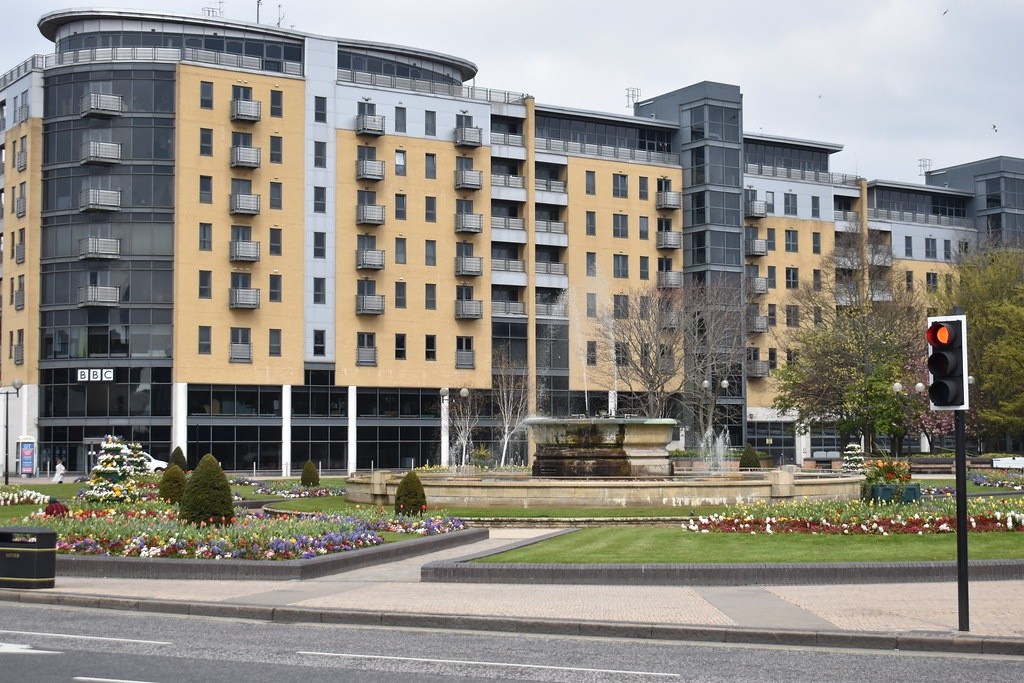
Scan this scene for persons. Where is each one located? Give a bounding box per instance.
[52,460,65,484]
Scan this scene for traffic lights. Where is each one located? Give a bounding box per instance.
[924,315,969,410]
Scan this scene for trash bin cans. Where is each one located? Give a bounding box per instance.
[0,526,57,589]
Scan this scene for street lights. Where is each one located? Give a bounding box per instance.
[0,379,22,485]
[721,378,729,429]
[915,382,926,438]
[460,388,469,417]
[702,379,711,430]
[892,382,902,456]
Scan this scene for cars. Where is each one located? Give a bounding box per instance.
[136,451,170,475]
